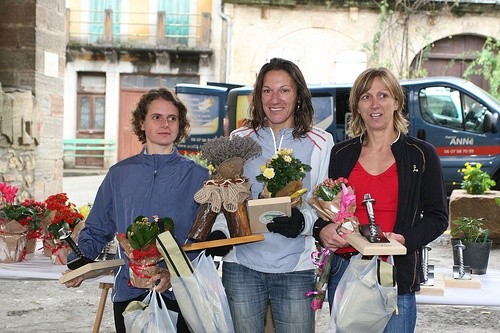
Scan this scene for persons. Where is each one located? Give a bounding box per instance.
[312,66,449,333]
[61,87,213,333]
[206,57,335,333]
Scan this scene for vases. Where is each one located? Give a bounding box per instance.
[0,211,85,264]
[450,189,500,246]
[121,243,160,289]
[279,179,303,208]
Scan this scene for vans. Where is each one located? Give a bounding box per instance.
[174,75,500,198]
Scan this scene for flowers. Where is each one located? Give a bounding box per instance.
[0,183,90,239]
[305,178,361,235]
[453,161,496,194]
[256,148,313,197]
[116,212,176,252]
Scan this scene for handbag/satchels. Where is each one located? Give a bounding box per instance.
[122,283,178,333]
[169,249,235,333]
[330,254,398,333]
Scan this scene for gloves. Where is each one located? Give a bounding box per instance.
[204,230,233,257]
[266,207,306,238]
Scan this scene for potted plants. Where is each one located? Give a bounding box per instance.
[450,217,493,275]
